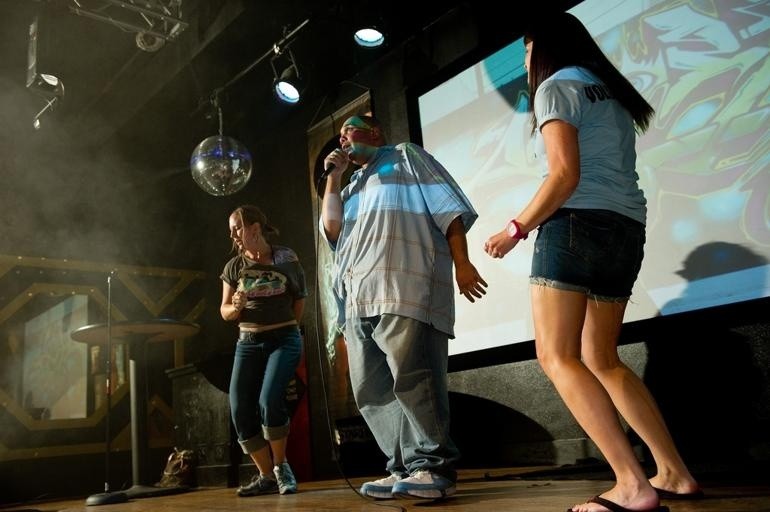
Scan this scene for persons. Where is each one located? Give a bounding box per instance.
[318,113,488,501]
[218,204,308,496]
[483,14,705,512]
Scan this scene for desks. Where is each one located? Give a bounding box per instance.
[69,312,204,500]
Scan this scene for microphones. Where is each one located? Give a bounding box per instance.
[107,268,118,280]
[321,149,346,179]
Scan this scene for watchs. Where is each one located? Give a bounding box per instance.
[505,219,530,240]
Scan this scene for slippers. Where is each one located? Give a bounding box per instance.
[652,484,702,501]
[567,495,669,512]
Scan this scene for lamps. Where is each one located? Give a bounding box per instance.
[269,37,318,105]
[352,0,388,48]
[26,2,73,100]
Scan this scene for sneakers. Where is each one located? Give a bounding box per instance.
[236,476,278,497]
[391,471,456,499]
[360,474,400,498]
[272,463,297,495]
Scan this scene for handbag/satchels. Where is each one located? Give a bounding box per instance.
[160,450,197,489]
